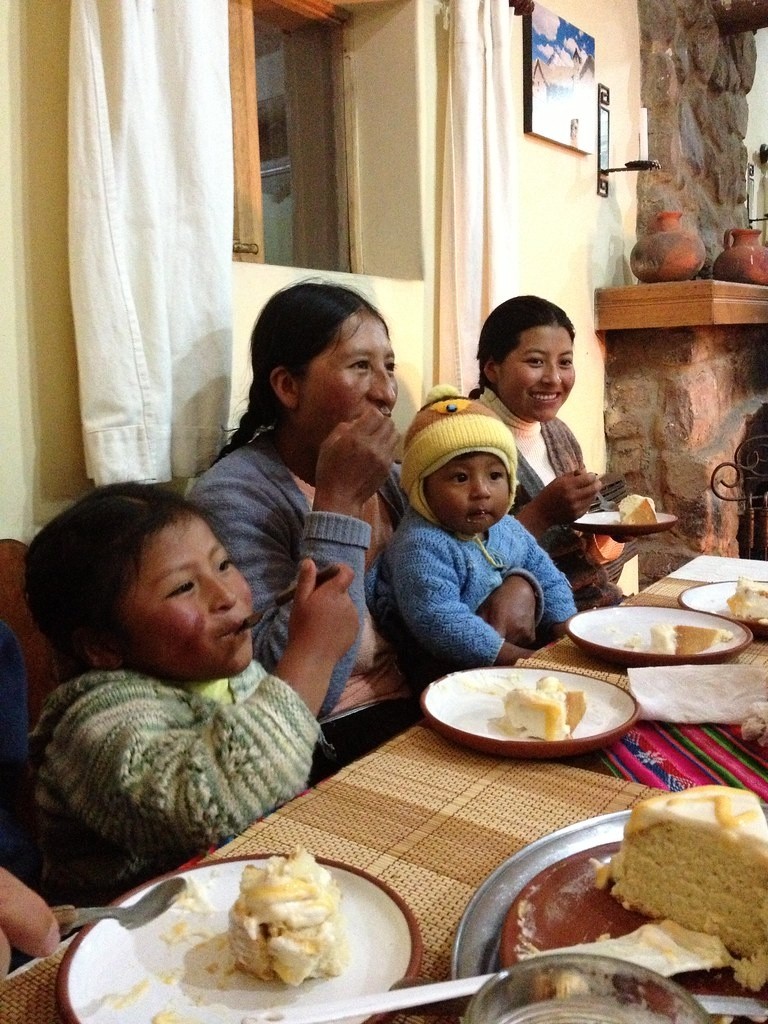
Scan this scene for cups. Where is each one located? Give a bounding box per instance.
[464,952,711,1024]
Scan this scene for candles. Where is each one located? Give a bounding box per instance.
[639,107,649,161]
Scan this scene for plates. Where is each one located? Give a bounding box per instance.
[500,840,768,1024]
[566,604,753,667]
[53,853,422,1024]
[419,665,642,759]
[677,581,768,638]
[571,511,678,536]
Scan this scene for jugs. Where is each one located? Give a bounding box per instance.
[713,228,768,286]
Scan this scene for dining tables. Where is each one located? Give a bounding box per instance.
[0,555,768,1024]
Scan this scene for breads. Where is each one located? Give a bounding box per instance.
[226,845,346,985]
[500,675,587,741]
[595,785,768,992]
[648,623,733,654]
[618,493,658,525]
[726,576,768,620]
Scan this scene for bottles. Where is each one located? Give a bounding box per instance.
[629,212,706,285]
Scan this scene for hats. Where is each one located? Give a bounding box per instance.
[400,383,518,538]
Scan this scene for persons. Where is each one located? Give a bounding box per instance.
[1,619,61,986]
[384,383,579,672]
[181,273,545,790]
[467,295,660,612]
[17,480,358,911]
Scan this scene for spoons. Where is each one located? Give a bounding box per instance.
[573,470,617,512]
[51,877,187,930]
[235,566,340,633]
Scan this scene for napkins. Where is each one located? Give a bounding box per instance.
[627,665,768,724]
